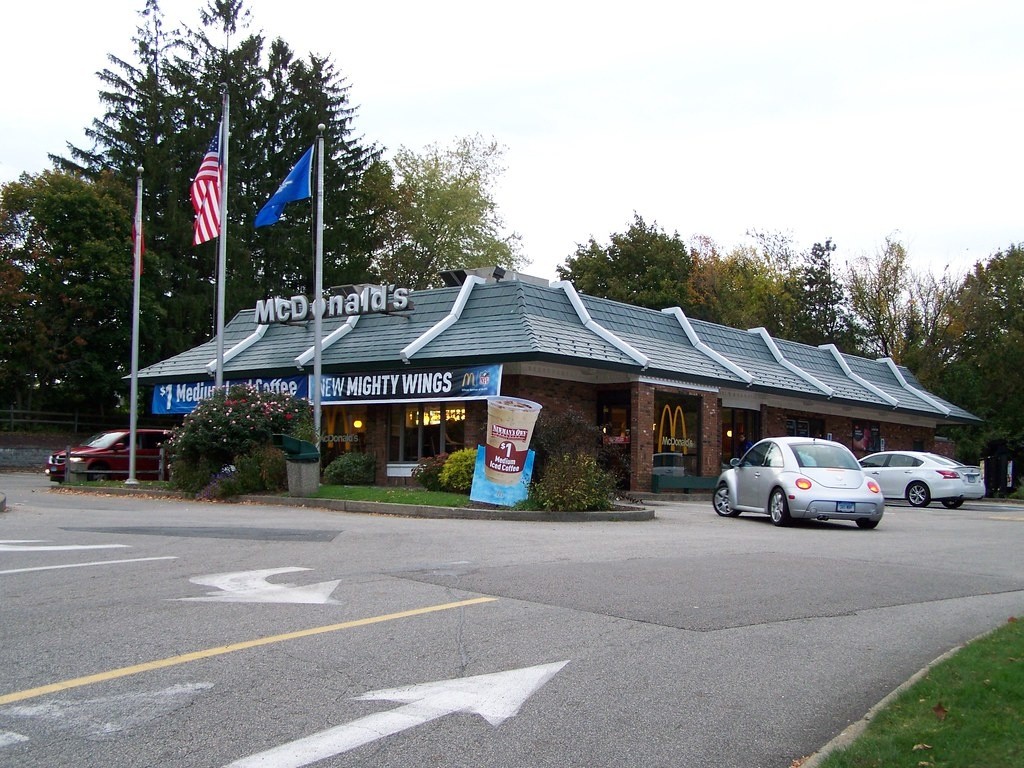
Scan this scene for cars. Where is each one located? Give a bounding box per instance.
[44,428,174,484]
[857,449,987,509]
[710,436,886,529]
[651,452,685,478]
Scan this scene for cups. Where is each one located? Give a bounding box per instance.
[485,397,543,486]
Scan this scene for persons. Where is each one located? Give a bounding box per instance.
[738,433,757,466]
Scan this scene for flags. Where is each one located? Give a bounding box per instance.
[132,196,145,282]
[191,94,226,246]
[254,143,314,228]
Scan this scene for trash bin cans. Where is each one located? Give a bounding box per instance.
[272,434,322,497]
